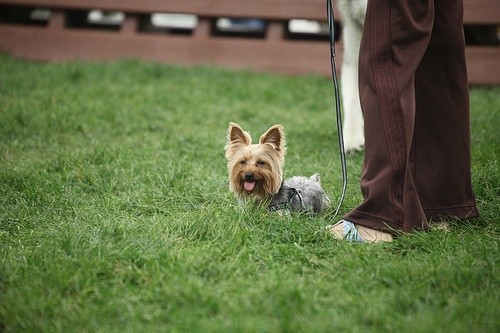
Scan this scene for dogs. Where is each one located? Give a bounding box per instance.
[224,120,331,223]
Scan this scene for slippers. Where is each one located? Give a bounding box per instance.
[325,219,393,243]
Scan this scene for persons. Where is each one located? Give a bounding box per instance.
[324,0,480,245]
